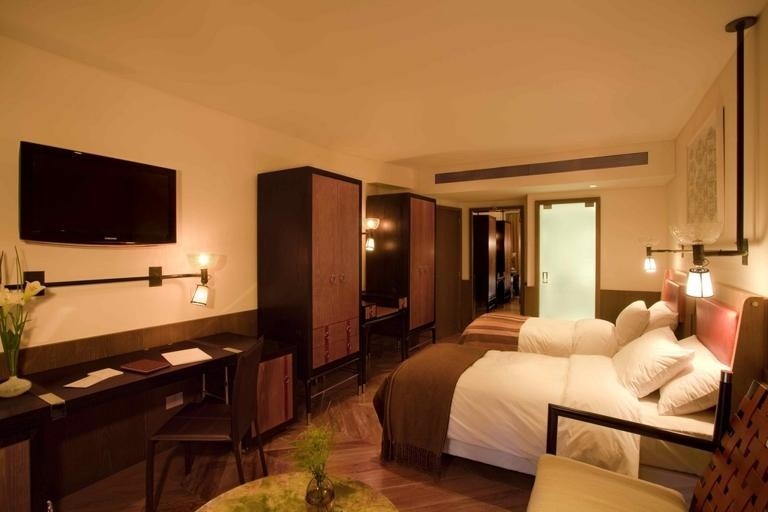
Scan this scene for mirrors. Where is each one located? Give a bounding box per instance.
[468,203,526,317]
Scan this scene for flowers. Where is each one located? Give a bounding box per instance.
[0,245,47,398]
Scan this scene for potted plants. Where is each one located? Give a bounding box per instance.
[295,423,338,512]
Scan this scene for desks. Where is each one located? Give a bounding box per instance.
[0,331,264,512]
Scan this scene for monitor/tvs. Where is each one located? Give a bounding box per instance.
[17,141,176,245]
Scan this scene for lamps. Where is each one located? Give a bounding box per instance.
[641,245,692,273]
[361,216,381,253]
[685,237,749,299]
[187,252,216,308]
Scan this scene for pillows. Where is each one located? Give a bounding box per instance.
[640,300,680,336]
[615,299,651,345]
[612,326,696,400]
[657,334,732,416]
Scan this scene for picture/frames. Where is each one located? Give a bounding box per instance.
[677,103,725,247]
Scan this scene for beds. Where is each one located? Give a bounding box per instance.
[370,280,768,502]
[456,267,695,353]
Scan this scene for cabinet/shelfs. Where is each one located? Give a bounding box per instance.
[255,164,364,426]
[364,191,438,362]
[208,350,295,446]
[473,214,498,313]
[495,220,514,306]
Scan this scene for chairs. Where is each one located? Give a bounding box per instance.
[360,298,409,394]
[524,369,768,512]
[144,335,269,512]
